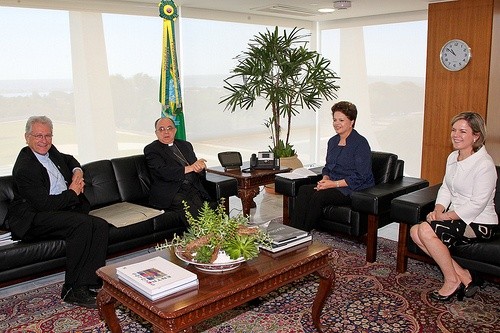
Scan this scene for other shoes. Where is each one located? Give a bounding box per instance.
[61,285,98,308]
[250,296,263,304]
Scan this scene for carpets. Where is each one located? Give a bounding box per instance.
[0,233,500,333]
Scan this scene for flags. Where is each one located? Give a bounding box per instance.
[158,19,186,141]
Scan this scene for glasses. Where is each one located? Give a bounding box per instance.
[27,134,53,140]
[155,127,175,132]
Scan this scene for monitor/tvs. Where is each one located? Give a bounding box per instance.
[218,152,242,166]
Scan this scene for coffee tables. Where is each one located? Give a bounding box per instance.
[95,235,335,333]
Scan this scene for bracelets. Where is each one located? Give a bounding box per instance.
[337,181,339,187]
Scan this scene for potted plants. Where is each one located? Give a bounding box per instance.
[221,25,339,194]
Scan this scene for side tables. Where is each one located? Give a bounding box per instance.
[205,161,293,222]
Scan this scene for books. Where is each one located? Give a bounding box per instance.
[116,256,199,301]
[256,220,312,253]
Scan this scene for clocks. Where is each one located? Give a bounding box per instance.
[440,40,471,71]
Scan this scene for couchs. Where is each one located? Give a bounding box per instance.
[275,149,427,262]
[0,153,237,283]
[391,166,500,280]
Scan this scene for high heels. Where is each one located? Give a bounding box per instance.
[431,282,465,302]
[462,268,484,296]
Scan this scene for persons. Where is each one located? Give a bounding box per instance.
[293,102,374,232]
[143,117,207,235]
[8,116,110,307]
[410,112,500,301]
